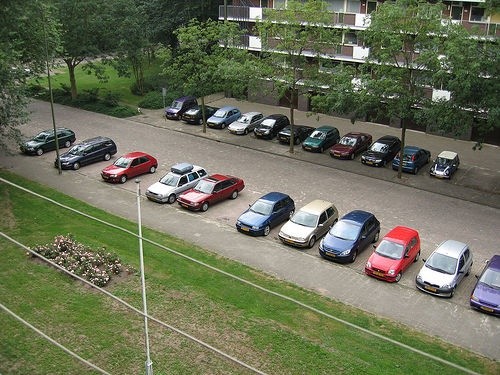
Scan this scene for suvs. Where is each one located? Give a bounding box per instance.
[54,135,118,171]
[165,97,199,120]
[19,127,76,156]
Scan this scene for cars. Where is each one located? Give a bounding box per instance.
[101,152,158,184]
[328,131,373,160]
[302,125,340,154]
[360,135,401,167]
[206,106,241,130]
[278,124,317,146]
[254,113,290,140]
[470,255,500,317]
[144,163,208,204]
[366,225,422,284]
[180,104,220,125]
[278,198,341,250]
[228,112,266,136]
[234,192,296,238]
[175,173,245,213]
[393,145,431,175]
[429,150,460,180]
[415,239,472,300]
[317,210,381,266]
